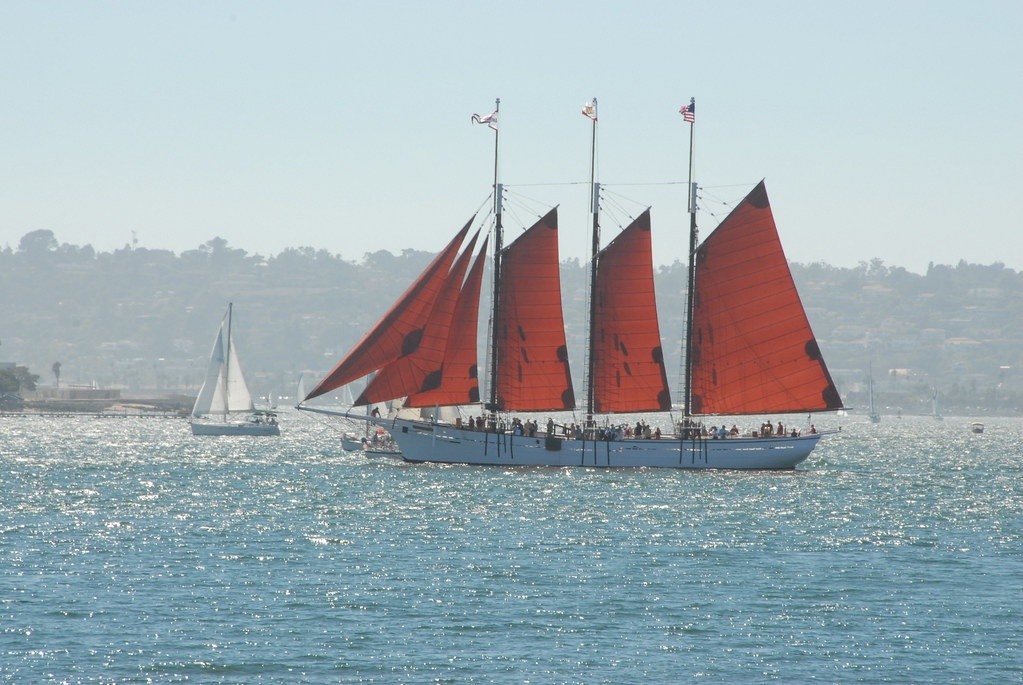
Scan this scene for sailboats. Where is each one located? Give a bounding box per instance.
[295,96,857,472]
[866,361,881,423]
[187,299,288,438]
[338,373,457,463]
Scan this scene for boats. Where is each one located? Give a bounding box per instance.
[970,421,986,434]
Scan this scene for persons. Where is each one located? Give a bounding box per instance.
[634,422,642,438]
[548,418,553,434]
[624,424,634,437]
[512,416,538,436]
[576,425,582,440]
[371,407,380,426]
[373,431,379,441]
[777,421,784,434]
[730,425,738,435]
[641,420,651,438]
[720,425,728,439]
[468,416,474,427]
[791,429,797,437]
[476,416,482,427]
[710,426,718,439]
[811,425,815,434]
[655,428,661,440]
[761,420,773,433]
